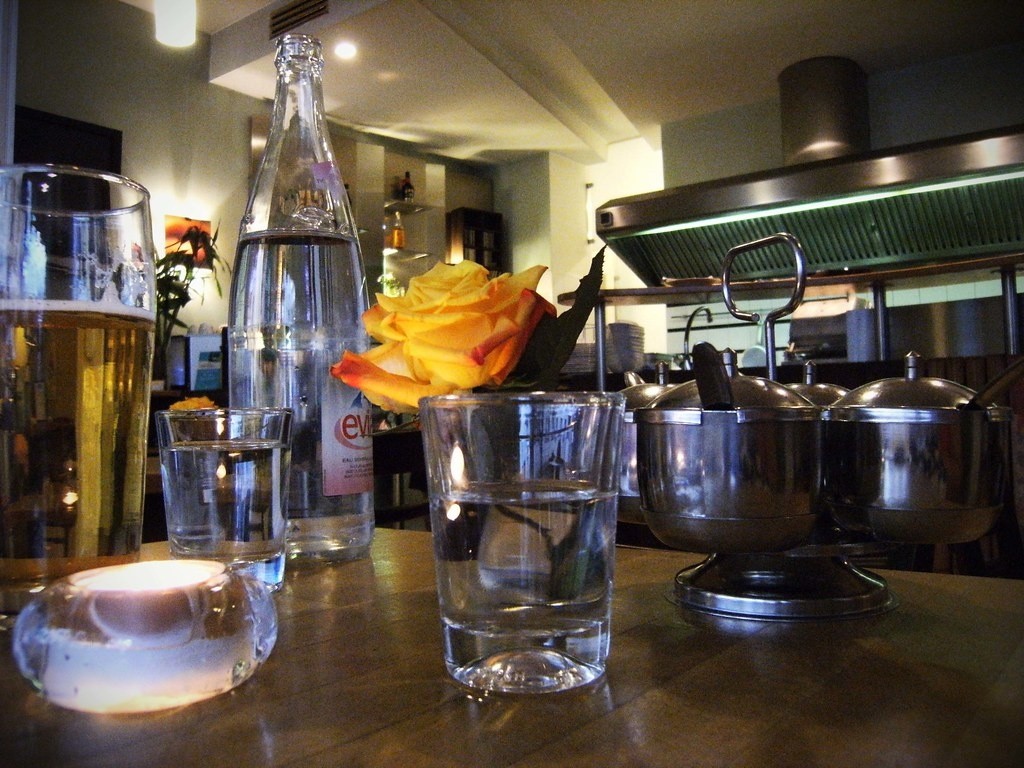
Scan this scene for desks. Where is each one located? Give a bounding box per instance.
[0,524,1024,768]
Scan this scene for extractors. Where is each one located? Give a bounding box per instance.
[595,123,1024,288]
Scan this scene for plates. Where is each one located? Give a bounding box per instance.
[560,342,597,372]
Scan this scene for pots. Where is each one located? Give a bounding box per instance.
[618,361,679,498]
[633,348,821,555]
[822,352,1014,545]
[784,360,850,411]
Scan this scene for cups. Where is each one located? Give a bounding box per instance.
[418,392,627,693]
[161,411,296,592]
[0,163,157,633]
[392,473,411,505]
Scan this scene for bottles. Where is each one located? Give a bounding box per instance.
[402,171,414,203]
[344,184,352,209]
[382,216,393,248]
[228,33,374,561]
[392,211,404,248]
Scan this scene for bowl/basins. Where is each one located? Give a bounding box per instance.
[605,323,644,371]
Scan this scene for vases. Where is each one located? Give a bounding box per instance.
[478,408,612,606]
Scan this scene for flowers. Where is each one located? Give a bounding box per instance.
[169,398,219,413]
[329,245,596,570]
